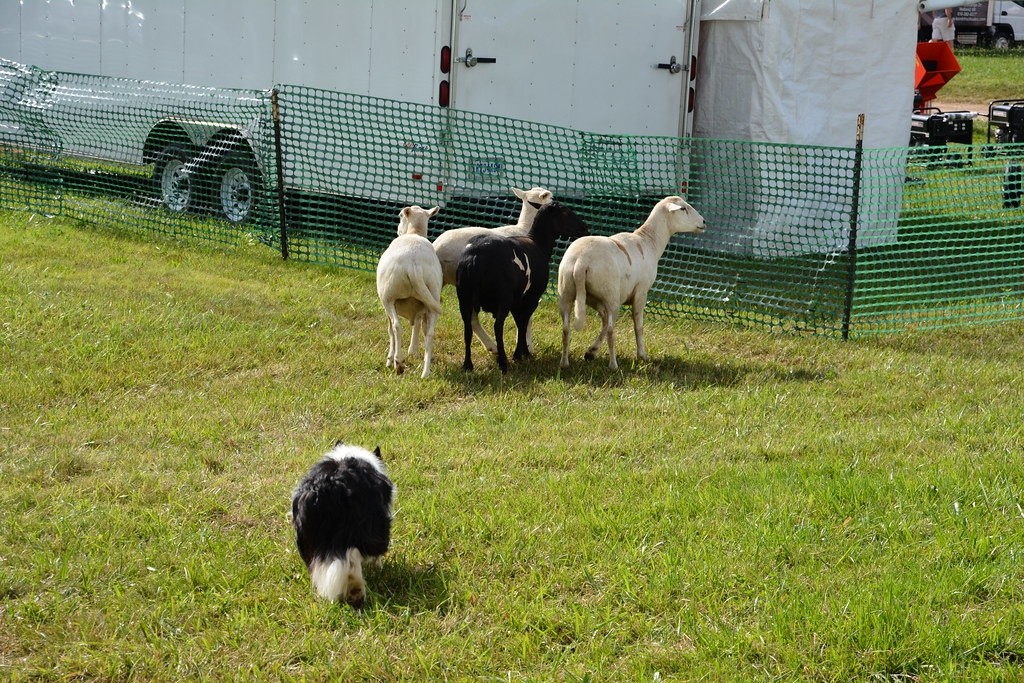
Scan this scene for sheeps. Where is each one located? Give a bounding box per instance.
[557,195,707,384]
[422,185,555,364]
[454,200,590,375]
[376,205,443,380]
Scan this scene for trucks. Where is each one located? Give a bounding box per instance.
[918,0,1024,55]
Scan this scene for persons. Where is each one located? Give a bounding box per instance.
[928,8,956,54]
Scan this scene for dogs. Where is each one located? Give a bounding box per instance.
[292,439,396,608]
